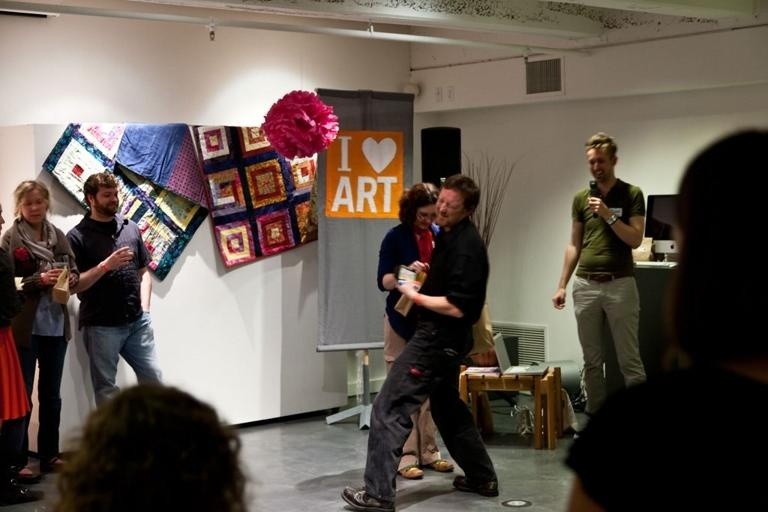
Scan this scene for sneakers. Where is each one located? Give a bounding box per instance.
[342,487,395,512]
[432,458,454,472]
[400,466,424,479]
[453,475,499,497]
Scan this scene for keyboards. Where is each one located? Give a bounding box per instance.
[634,260,676,266]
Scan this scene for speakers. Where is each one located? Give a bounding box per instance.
[420,126,461,180]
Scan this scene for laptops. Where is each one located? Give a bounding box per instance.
[492,333,549,376]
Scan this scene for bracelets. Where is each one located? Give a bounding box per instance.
[413,293,422,304]
[607,214,617,225]
[99,261,109,272]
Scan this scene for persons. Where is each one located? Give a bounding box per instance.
[0,180,80,483]
[552,130,647,415]
[340,175,499,512]
[66,173,162,406]
[52,382,249,512]
[565,123,768,511]
[0,204,44,507]
[377,182,454,479]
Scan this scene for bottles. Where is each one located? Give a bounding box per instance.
[397,279,422,292]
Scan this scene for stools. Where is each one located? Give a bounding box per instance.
[456,365,563,453]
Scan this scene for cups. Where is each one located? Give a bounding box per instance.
[123,249,134,263]
[52,262,66,269]
[417,272,427,283]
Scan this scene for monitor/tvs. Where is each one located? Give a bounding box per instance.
[644,194,681,254]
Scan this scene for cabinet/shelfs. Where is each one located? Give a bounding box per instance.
[605,265,681,396]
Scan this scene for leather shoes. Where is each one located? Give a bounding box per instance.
[1,468,43,506]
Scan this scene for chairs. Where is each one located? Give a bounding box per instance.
[489,333,589,434]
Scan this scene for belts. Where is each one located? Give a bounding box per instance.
[579,272,625,283]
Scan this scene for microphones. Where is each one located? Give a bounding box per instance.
[589,181,599,219]
